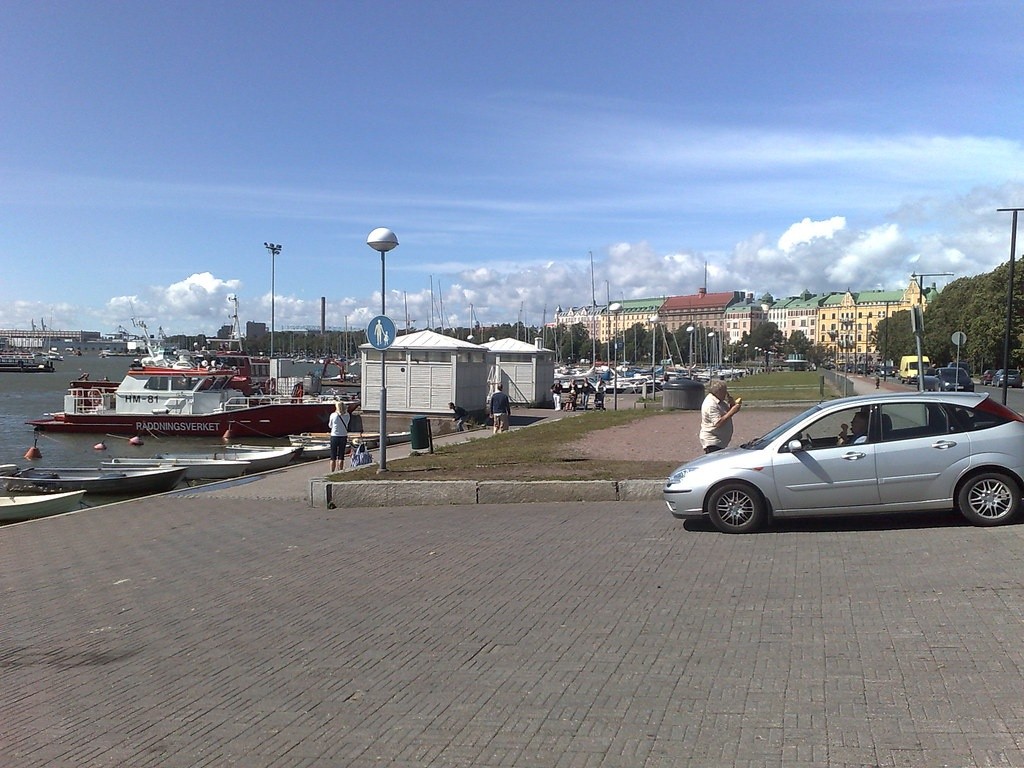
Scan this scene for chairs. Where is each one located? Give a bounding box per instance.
[882,413,893,439]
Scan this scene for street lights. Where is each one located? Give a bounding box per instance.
[708,332,715,390]
[650,316,660,400]
[755,346,759,362]
[996,208,1024,409]
[361,227,401,484]
[609,303,624,410]
[743,343,748,373]
[686,325,695,382]
[263,240,282,358]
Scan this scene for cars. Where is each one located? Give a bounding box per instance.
[979,370,999,386]
[992,369,1023,388]
[823,355,974,393]
[662,390,1024,534]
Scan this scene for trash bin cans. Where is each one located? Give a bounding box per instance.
[409,414,430,449]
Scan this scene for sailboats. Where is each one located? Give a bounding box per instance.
[514,250,751,394]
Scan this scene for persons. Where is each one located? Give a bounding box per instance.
[927,363,936,391]
[596,378,606,408]
[581,378,597,411]
[663,373,669,382]
[875,373,880,389]
[490,384,511,434]
[699,380,741,455]
[329,402,350,472]
[1017,365,1022,375]
[569,378,580,409]
[449,403,471,431]
[348,439,361,466]
[551,380,563,411]
[807,412,868,448]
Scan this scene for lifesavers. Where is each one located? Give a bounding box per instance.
[88,389,102,403]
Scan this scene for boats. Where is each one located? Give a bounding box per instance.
[100,457,252,479]
[286,430,413,450]
[159,447,303,473]
[0,347,65,372]
[0,489,89,523]
[24,300,362,439]
[321,372,362,388]
[0,463,189,495]
[98,351,362,366]
[225,443,352,460]
